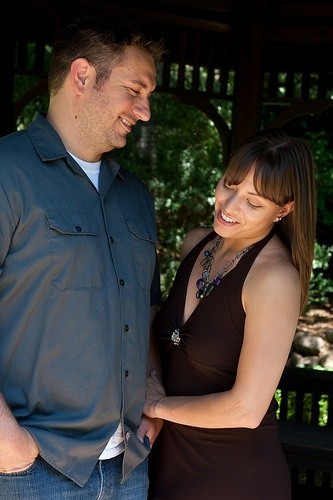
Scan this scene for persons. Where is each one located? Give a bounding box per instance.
[0,9,167,500]
[144,127,316,500]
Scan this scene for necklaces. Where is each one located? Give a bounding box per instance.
[195,234,258,300]
